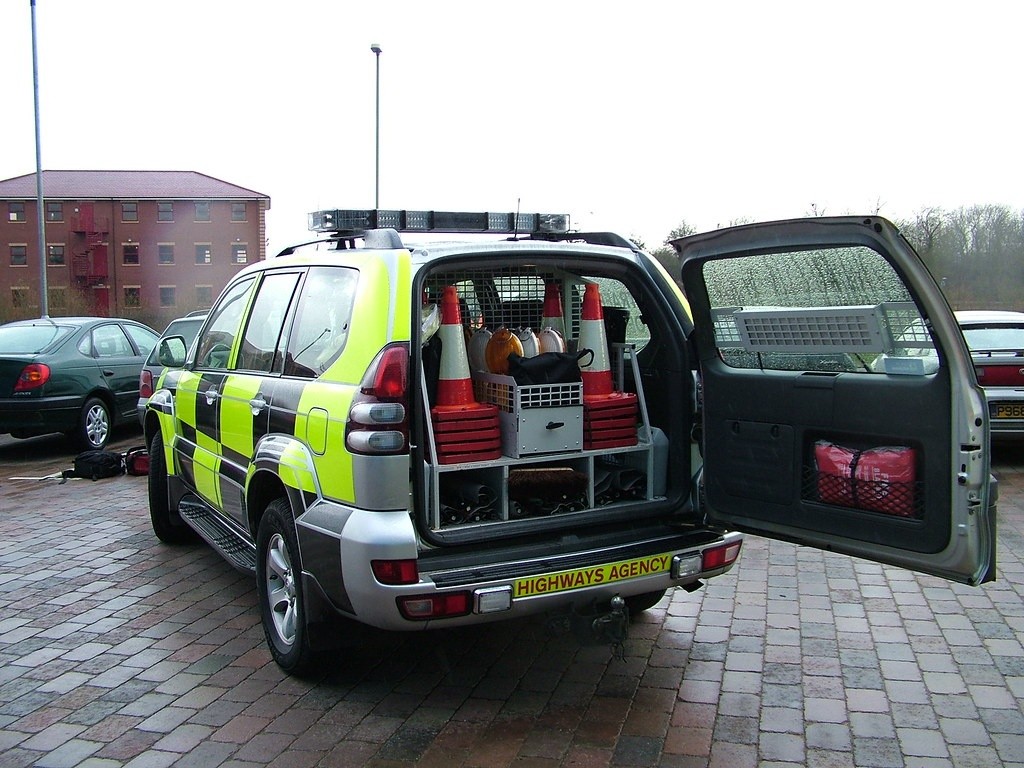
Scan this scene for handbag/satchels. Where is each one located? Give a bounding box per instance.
[125,446,150,476]
[506,348,594,409]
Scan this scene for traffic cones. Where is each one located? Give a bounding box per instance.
[430,283,640,466]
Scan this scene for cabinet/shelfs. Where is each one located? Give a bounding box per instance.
[421,341,655,534]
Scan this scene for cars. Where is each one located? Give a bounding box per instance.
[0,317,162,452]
[849,310,1024,460]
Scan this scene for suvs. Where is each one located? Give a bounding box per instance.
[144,210,997,677]
[136,309,211,426]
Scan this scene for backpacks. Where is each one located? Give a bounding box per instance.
[58,450,126,484]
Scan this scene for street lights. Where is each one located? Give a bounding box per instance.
[371,43,382,211]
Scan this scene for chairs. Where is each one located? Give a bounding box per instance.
[95,336,117,358]
